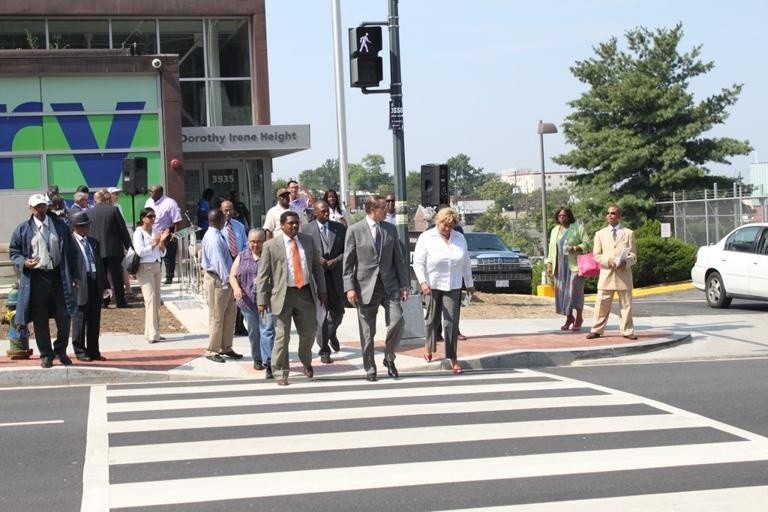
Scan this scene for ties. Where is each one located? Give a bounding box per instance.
[375,223,381,257]
[290,239,304,290]
[82,238,97,280]
[322,225,328,272]
[227,222,238,257]
[40,223,49,266]
[612,228,617,242]
[221,235,226,242]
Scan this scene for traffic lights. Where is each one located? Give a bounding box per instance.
[345,22,392,90]
[347,26,386,88]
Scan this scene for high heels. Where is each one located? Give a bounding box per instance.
[573,319,583,331]
[424,342,432,362]
[450,361,462,373]
[561,317,575,331]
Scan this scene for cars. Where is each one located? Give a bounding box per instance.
[463,232,531,292]
[690,223,768,309]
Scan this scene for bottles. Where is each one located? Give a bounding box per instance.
[563,240,569,255]
[463,292,471,304]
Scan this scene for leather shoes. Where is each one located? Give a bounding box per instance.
[367,373,376,381]
[330,336,339,351]
[277,379,288,385]
[164,278,172,284]
[624,334,637,340]
[90,356,105,361]
[587,333,600,339]
[220,351,243,359]
[265,367,274,379]
[56,352,72,365]
[383,358,398,378]
[304,365,313,378]
[457,334,467,340]
[321,352,330,363]
[76,353,92,362]
[254,360,262,370]
[206,355,225,363]
[40,356,53,368]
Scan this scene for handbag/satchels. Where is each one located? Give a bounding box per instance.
[420,290,435,326]
[121,229,144,275]
[198,206,212,219]
[577,242,600,277]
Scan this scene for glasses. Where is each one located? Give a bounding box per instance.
[386,200,395,203]
[146,215,156,218]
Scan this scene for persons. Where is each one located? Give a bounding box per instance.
[413,206,473,374]
[300,202,349,363]
[384,193,395,222]
[134,208,170,344]
[220,199,250,336]
[266,187,308,233]
[343,195,409,382]
[324,188,345,222]
[547,205,590,332]
[87,189,134,309]
[107,186,124,215]
[50,195,69,217]
[9,195,81,368]
[202,209,243,365]
[196,188,216,240]
[101,189,133,299]
[232,191,254,228]
[70,212,114,363]
[427,203,468,342]
[255,212,318,386]
[228,227,276,378]
[586,205,637,341]
[44,185,59,203]
[77,186,94,211]
[287,181,317,211]
[71,192,90,210]
[145,185,182,284]
[264,228,275,238]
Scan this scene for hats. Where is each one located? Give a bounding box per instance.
[107,187,122,193]
[277,188,291,197]
[29,194,48,207]
[71,212,92,226]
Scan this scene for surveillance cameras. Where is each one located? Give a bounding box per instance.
[152,58,161,69]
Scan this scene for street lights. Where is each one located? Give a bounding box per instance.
[537,114,558,259]
[539,120,560,256]
